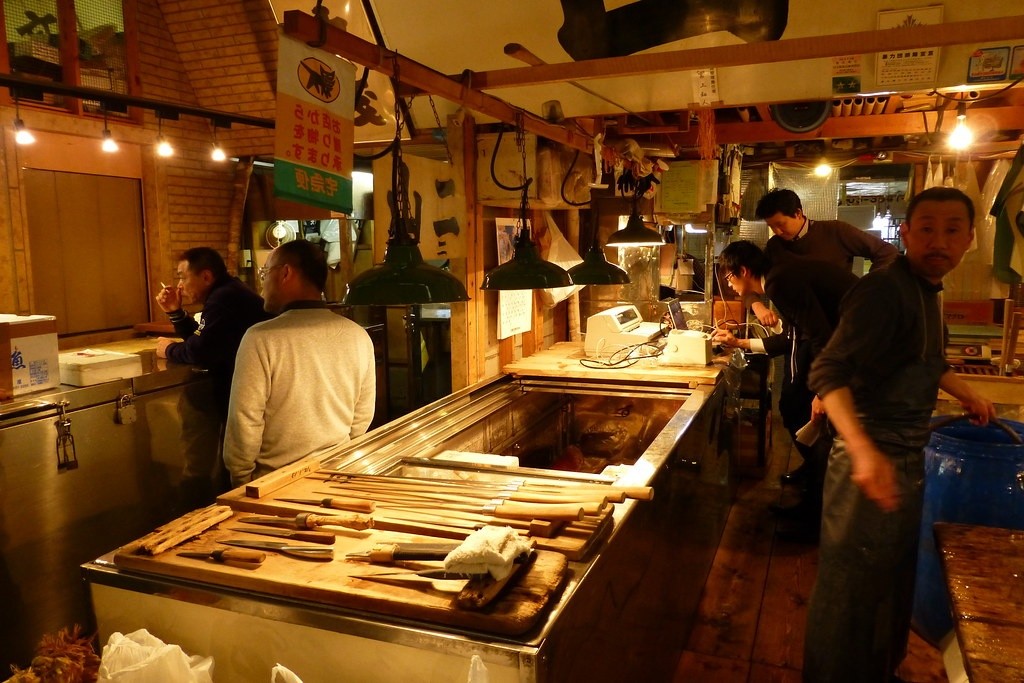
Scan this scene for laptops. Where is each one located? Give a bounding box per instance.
[668,297,726,355]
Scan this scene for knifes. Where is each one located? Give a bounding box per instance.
[228,528,335,544]
[237,513,374,530]
[347,565,484,581]
[217,541,334,559]
[176,548,266,563]
[274,499,376,513]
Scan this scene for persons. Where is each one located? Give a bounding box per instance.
[222,240,376,488]
[802,187,998,683]
[710,240,791,357]
[156,247,279,421]
[743,188,899,486]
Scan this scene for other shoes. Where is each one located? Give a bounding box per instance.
[780,472,804,486]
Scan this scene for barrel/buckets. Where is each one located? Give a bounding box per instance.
[910,414,1024,649]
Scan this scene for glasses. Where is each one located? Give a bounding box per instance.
[723,265,740,281]
[257,263,284,281]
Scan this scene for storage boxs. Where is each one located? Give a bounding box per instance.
[0,313,60,400]
[59,349,143,387]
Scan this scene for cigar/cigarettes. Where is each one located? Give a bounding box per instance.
[160,281,170,294]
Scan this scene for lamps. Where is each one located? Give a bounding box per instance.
[479,120,574,290]
[605,179,666,246]
[568,155,630,285]
[343,67,472,304]
